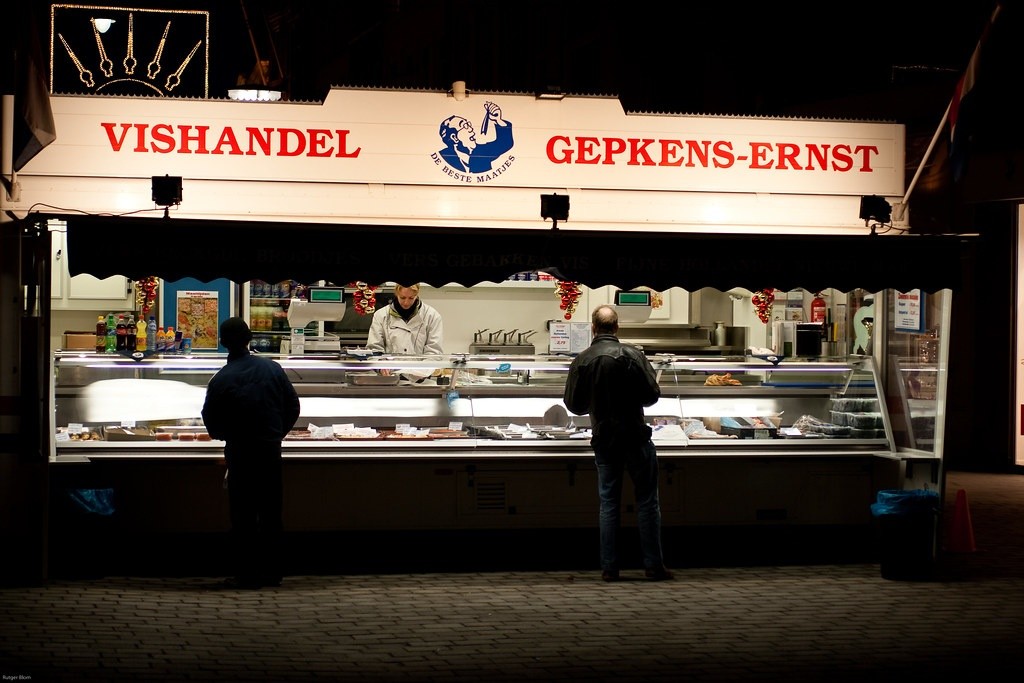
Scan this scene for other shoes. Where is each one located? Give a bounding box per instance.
[222,575,283,590]
[601,567,621,582]
[644,565,673,582]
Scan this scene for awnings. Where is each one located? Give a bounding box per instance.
[20,211,980,299]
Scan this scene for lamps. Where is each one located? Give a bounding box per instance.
[539,194,570,230]
[152,174,184,222]
[860,194,892,236]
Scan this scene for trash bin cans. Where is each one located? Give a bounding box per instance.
[54,487,115,571]
[877,489,939,581]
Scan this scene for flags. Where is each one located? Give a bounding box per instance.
[947,18,989,145]
[11,0,56,173]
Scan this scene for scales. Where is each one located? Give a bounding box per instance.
[280,287,346,354]
[603,291,652,323]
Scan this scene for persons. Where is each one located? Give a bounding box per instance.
[564,305,675,582]
[202,317,300,591]
[367,283,446,382]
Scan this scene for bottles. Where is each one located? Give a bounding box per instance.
[95,314,175,354]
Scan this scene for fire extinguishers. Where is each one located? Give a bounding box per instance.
[810,289,829,323]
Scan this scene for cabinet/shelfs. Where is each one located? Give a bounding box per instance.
[46,217,136,311]
